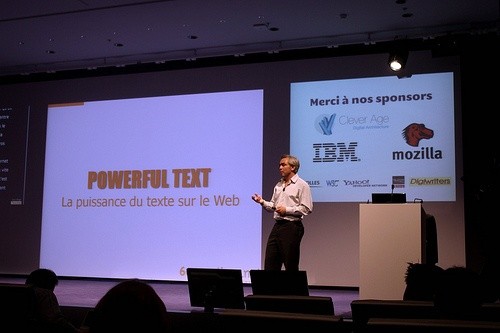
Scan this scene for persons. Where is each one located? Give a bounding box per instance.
[251,155,313,268]
[24,269,79,333]
[403,263,445,301]
[433,267,483,321]
[89,280,167,333]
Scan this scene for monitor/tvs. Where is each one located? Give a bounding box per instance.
[372,192,406,204]
[187,267,245,314]
[250,269,310,297]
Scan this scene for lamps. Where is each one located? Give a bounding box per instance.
[387,44,409,71]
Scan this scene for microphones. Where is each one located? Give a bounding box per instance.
[390,185,395,203]
